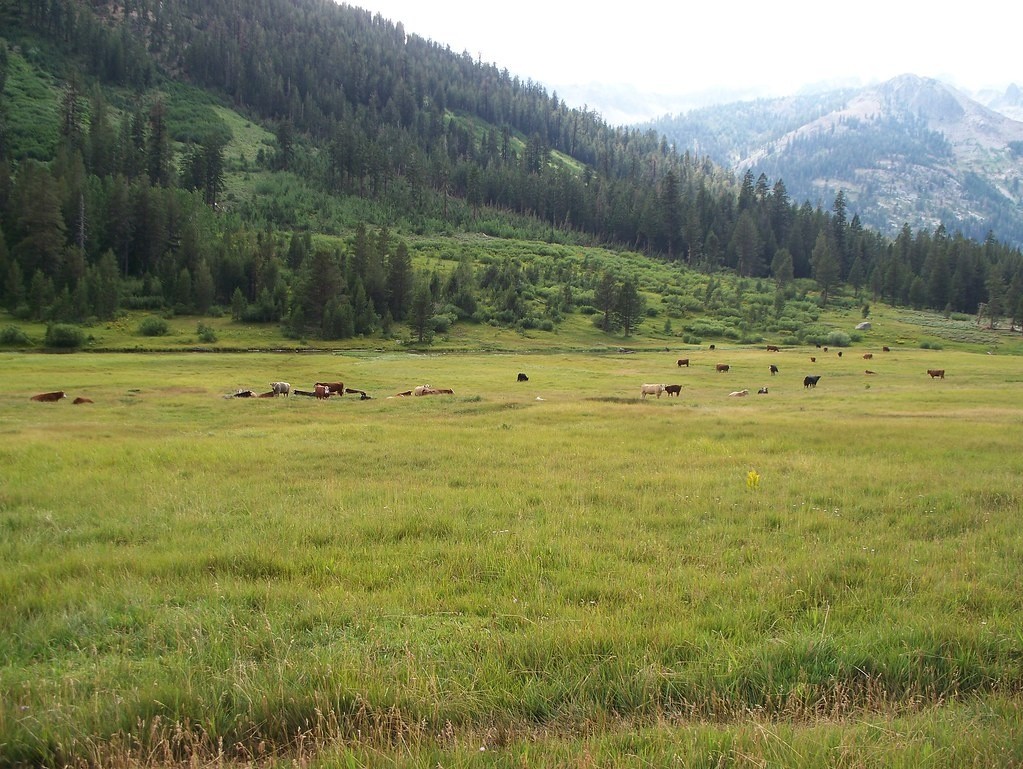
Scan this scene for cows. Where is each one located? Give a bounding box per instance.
[865,370,874,374]
[517,373,528,382]
[676,359,689,367]
[224,388,276,399]
[928,370,944,379]
[768,365,778,376]
[863,346,890,360]
[717,363,732,373]
[71,398,93,404]
[709,345,715,350]
[665,385,682,397]
[297,380,371,400]
[728,387,768,397]
[30,390,66,402]
[767,345,779,352]
[804,376,821,388]
[811,343,842,362]
[641,383,666,399]
[270,382,290,397]
[387,384,454,400]
